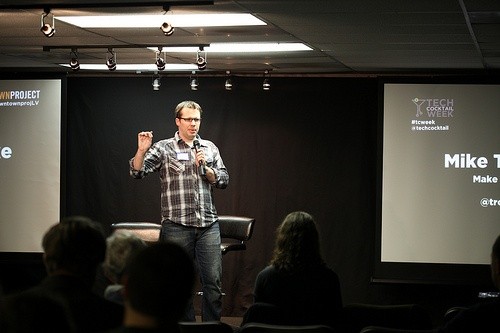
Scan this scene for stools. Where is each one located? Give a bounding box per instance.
[197,216,254,295]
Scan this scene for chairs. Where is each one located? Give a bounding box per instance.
[176,302,469,333]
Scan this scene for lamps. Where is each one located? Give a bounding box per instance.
[69,48,80,73]
[40,8,56,38]
[105,48,117,71]
[263,70,272,91]
[224,71,233,91]
[189,71,200,91]
[196,46,208,71]
[151,72,161,91]
[154,46,167,71]
[161,6,175,36]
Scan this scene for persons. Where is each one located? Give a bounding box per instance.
[129,101,228,322]
[15,217,196,333]
[254,212,346,333]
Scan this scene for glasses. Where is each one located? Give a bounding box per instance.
[178,116,201,123]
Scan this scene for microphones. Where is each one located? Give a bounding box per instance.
[193,140,206,178]
